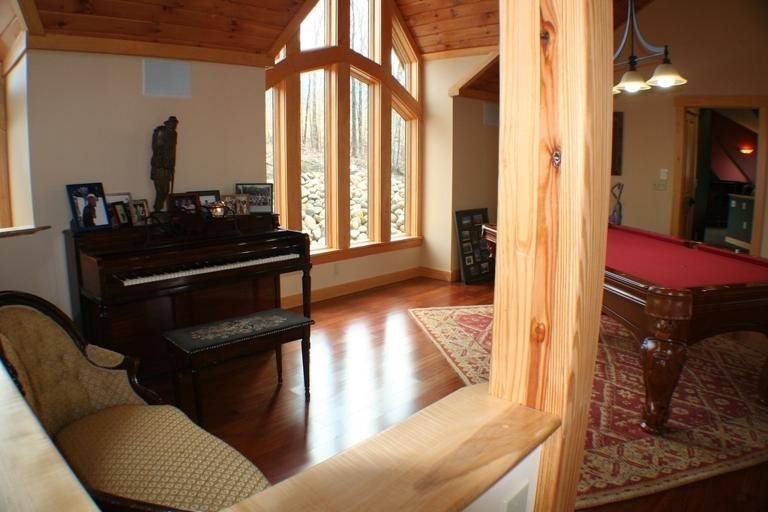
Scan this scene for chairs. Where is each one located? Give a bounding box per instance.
[0,290,275,511]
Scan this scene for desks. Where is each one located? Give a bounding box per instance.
[480,222,768,435]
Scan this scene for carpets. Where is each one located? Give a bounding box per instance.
[406,303,767,509]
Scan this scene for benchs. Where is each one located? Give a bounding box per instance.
[161,307,316,427]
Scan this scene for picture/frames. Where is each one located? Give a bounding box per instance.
[65,182,274,233]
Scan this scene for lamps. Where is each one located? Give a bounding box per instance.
[612,1,689,95]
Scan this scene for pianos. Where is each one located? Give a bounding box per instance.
[63,212,312,348]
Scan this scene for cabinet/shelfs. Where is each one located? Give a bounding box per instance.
[725,192,755,253]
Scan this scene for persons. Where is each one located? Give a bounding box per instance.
[80,194,99,227]
[184,198,196,213]
[174,199,181,212]
[149,116,180,211]
[224,198,248,215]
[139,206,145,220]
[243,191,270,206]
[201,199,211,209]
[179,199,187,212]
